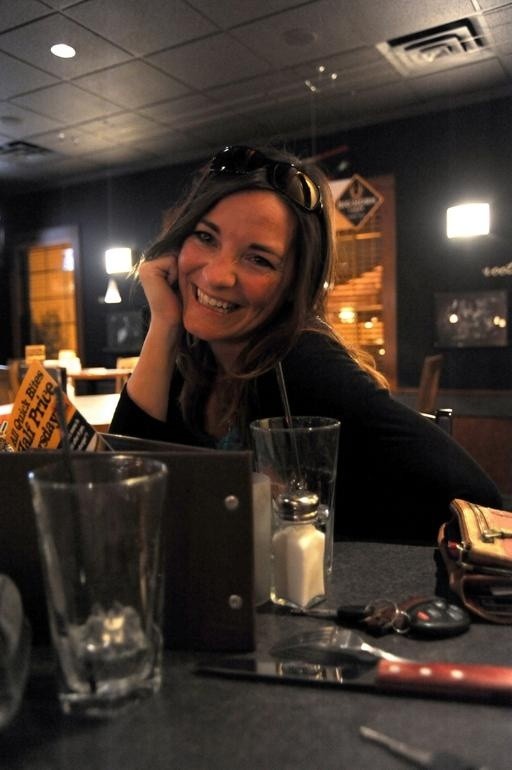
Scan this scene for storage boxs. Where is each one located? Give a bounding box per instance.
[0,432,258,654]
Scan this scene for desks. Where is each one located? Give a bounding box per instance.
[1,391,122,434]
[66,368,133,397]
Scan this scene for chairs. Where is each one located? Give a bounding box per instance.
[116,356,139,395]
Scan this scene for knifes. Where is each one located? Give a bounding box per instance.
[194,657,510,703]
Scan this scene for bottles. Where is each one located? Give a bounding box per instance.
[272,489,327,610]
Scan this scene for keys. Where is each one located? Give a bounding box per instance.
[290,599,469,639]
[359,723,480,769]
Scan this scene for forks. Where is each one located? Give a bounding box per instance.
[271,625,407,663]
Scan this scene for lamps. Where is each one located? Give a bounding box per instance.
[104,162,122,304]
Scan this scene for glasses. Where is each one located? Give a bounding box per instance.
[206,143,322,211]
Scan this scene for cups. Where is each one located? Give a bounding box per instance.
[27,457,169,717]
[250,418,337,608]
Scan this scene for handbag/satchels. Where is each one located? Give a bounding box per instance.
[433,496,512,625]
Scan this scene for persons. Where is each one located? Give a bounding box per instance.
[109,145,504,547]
